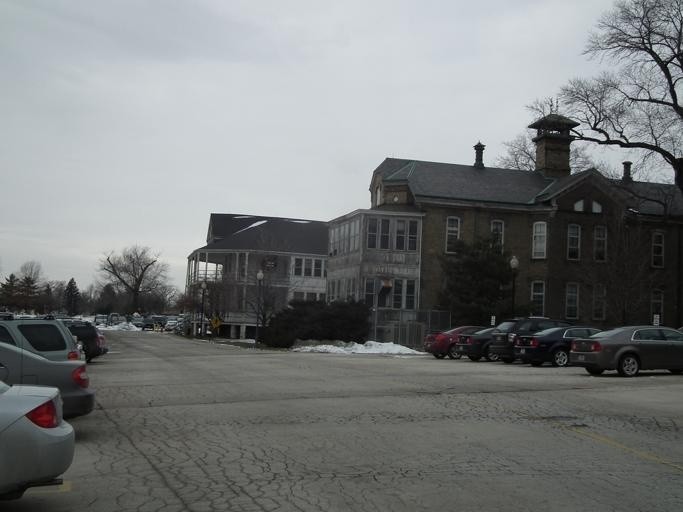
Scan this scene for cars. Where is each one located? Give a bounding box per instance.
[0,316,86,363]
[0,341,96,420]
[61,317,109,364]
[424,316,683,376]
[0,381,78,500]
